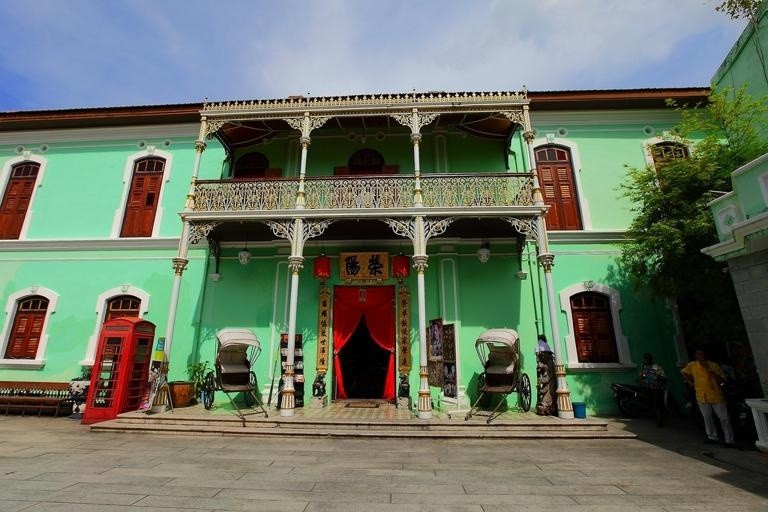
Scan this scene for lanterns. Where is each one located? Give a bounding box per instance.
[391,250,410,284]
[314,249,331,286]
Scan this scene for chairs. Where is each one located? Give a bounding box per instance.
[484,350,515,386]
[219,350,251,385]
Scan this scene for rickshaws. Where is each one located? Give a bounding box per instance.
[461,328,532,423]
[201,327,269,423]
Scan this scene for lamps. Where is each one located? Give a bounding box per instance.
[476,222,491,264]
[238,222,251,265]
[313,233,332,280]
[391,235,410,279]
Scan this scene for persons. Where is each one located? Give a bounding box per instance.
[680,348,737,447]
[430,323,443,355]
[635,354,670,428]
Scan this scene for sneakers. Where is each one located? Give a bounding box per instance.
[724,442,737,449]
[703,439,720,445]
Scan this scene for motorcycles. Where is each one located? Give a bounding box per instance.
[611,382,679,421]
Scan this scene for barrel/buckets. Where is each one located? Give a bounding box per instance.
[572,401,588,419]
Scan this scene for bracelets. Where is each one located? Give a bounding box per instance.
[707,369,711,373]
